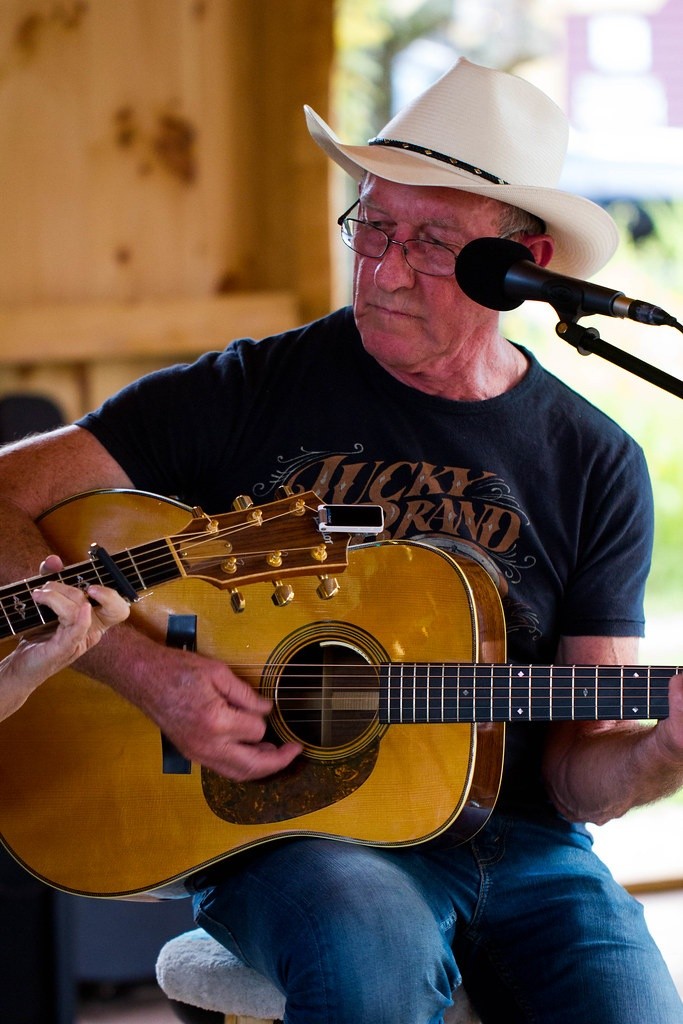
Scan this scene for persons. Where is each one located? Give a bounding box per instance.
[0,60,683,1024]
[0,554,133,727]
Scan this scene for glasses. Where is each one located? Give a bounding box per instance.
[337,199,529,277]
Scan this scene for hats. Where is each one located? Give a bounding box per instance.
[304,56,619,281]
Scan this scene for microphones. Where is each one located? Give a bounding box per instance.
[456,236,670,325]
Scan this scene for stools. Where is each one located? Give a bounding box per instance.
[155,929,288,1024]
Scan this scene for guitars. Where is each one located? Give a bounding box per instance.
[0,482,352,646]
[0,487,683,905]
